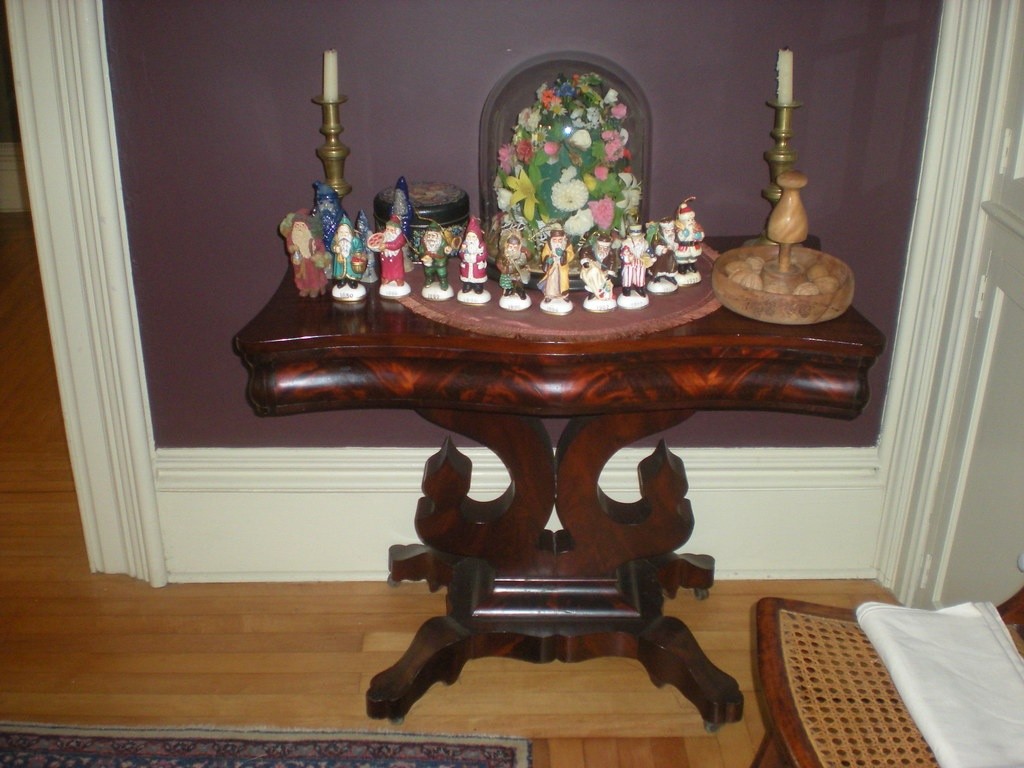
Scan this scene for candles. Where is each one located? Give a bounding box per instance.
[777,46,794,105]
[322,46,339,103]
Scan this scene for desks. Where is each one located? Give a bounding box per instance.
[232,233,887,733]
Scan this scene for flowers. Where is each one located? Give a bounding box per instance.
[494,73,642,251]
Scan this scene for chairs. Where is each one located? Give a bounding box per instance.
[749,582,1024,768]
